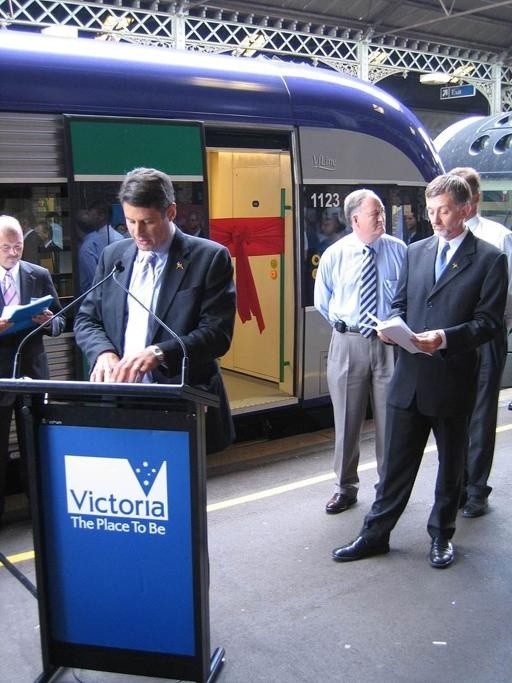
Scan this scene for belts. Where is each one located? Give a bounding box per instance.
[334,322,360,333]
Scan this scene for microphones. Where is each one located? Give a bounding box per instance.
[112,266,190,385]
[13,259,122,379]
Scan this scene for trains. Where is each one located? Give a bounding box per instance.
[2,25,512,468]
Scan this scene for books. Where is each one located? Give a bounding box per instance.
[0,295,53,336]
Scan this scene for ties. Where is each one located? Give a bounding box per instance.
[359,246,378,341]
[123,254,156,384]
[3,271,19,305]
[435,242,451,284]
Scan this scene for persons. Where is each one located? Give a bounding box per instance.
[317,216,345,258]
[403,208,430,245]
[73,168,237,456]
[21,199,209,304]
[313,188,408,515]
[331,174,508,569]
[0,214,68,515]
[449,166,511,517]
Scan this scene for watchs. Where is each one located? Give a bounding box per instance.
[146,344,166,363]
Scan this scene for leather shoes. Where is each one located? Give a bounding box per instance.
[462,494,487,518]
[428,535,455,566]
[331,535,391,560]
[326,492,357,513]
